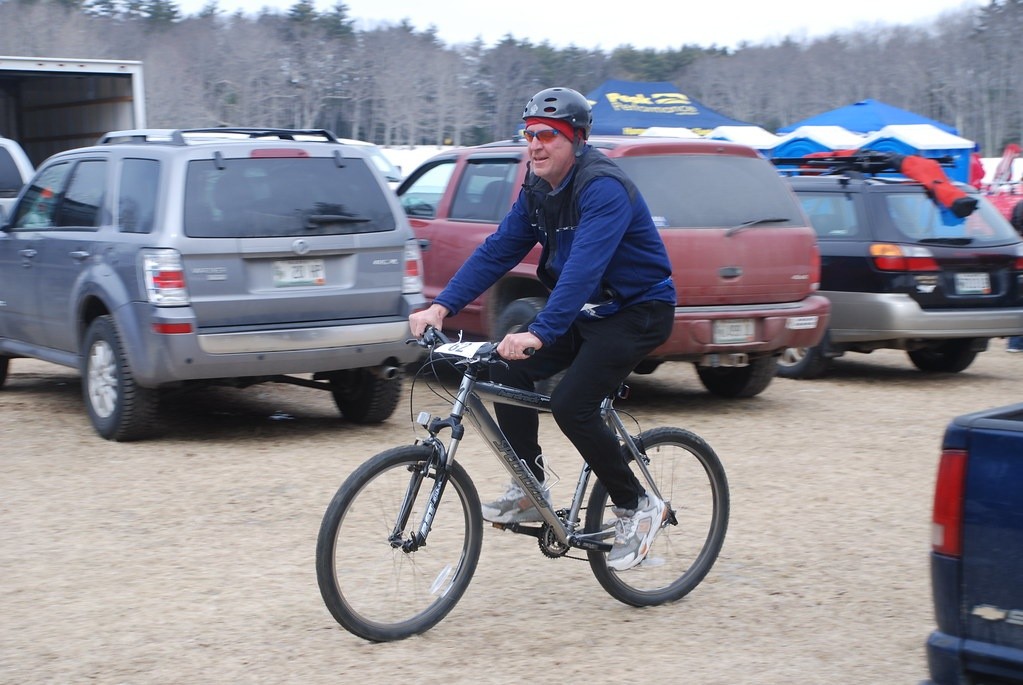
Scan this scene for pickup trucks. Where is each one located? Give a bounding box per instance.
[925,402,1023,685]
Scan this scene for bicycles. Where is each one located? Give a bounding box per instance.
[315,322,731,643]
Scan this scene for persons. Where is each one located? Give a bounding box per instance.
[407,86,675,568]
[1006,199,1023,353]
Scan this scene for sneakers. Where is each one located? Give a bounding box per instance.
[606,493,668,572]
[481,476,553,524]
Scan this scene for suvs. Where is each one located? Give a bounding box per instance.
[764,155,1023,379]
[0,136,50,229]
[0,128,429,439]
[390,135,829,400]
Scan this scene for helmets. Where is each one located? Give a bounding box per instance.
[522,87,593,140]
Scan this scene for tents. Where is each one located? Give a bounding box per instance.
[515,75,784,151]
[758,99,980,186]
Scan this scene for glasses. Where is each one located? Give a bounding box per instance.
[523,129,560,143]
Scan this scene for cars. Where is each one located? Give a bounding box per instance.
[295,136,507,198]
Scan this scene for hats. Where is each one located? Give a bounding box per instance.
[526,109,585,144]
